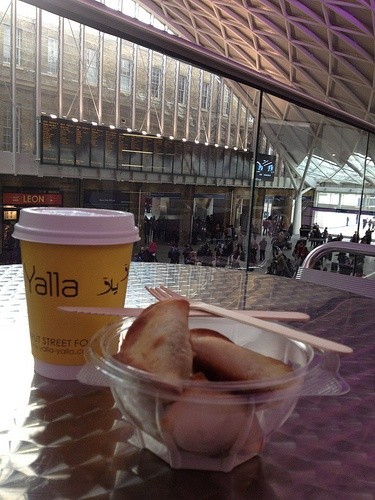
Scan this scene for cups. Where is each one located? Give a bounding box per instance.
[12,207,141,380]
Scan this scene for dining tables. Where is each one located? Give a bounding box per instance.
[0,261,375,500]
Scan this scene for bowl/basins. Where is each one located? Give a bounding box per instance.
[77,317,350,473]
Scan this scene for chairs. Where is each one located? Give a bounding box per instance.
[296,242,375,299]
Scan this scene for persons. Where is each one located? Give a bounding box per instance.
[0,197,375,280]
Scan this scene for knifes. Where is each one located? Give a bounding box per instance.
[57,306,311,319]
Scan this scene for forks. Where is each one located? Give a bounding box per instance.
[145,285,353,353]
